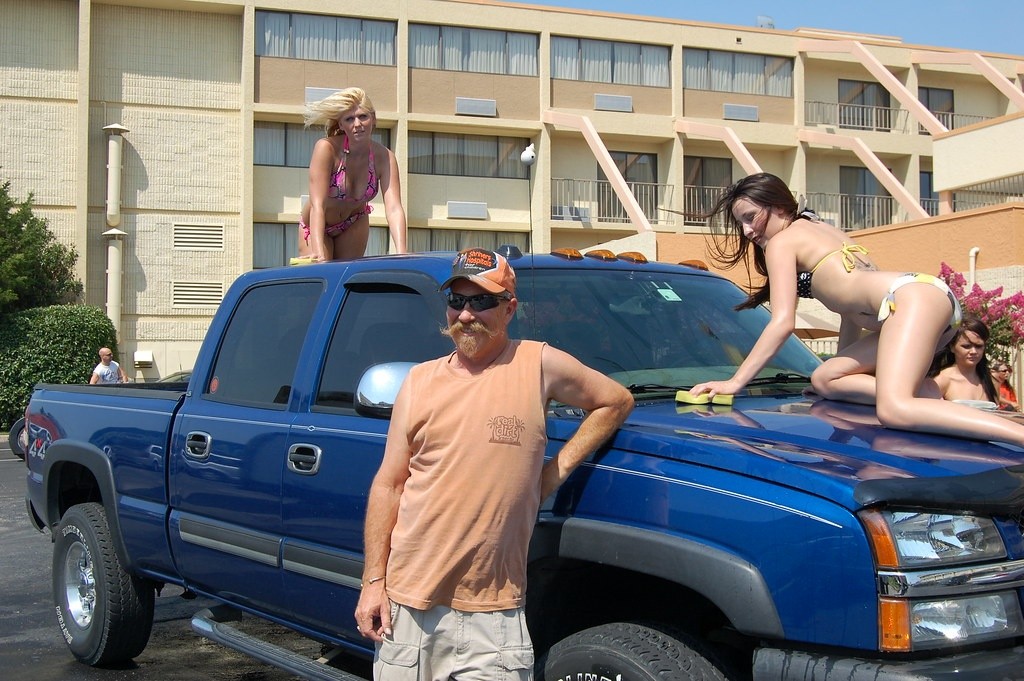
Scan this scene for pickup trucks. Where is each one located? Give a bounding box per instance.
[22,244,1024,681]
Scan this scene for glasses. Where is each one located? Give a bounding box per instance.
[103,353,112,356]
[441,290,514,313]
[998,369,1008,373]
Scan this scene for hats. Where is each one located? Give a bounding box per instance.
[438,246,516,302]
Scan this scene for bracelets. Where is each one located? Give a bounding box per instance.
[359,576,387,587]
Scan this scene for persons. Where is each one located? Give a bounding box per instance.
[298,87,407,261]
[89,348,128,385]
[994,362,1021,413]
[688,172,1024,450]
[677,399,1023,483]
[353,249,634,679]
[933,318,999,411]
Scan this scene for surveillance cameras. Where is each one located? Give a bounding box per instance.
[521,151,535,165]
